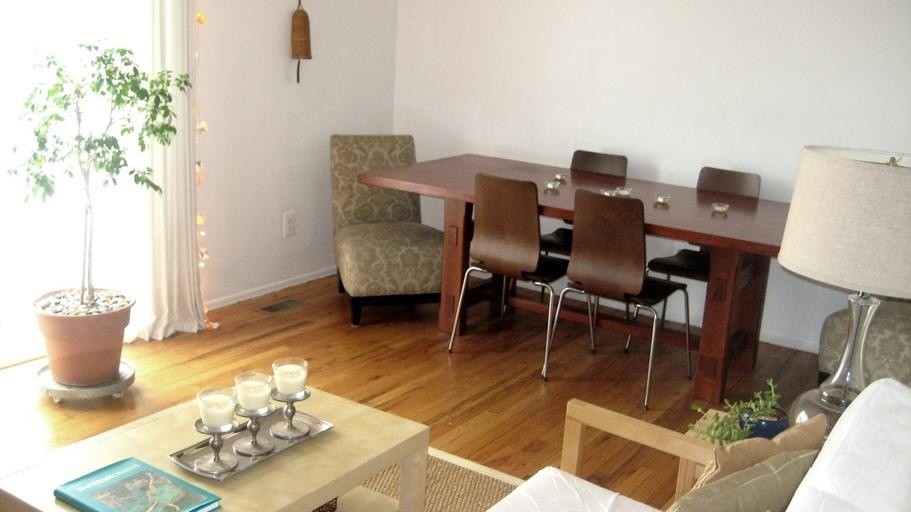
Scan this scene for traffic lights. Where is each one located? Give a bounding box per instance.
[664,414,827,512]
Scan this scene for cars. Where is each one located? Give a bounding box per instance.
[545,171,731,214]
[233,372,273,415]
[271,356,309,396]
[197,385,237,433]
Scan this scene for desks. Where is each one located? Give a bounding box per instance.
[353,154,797,405]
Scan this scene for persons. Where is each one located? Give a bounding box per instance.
[93,473,180,512]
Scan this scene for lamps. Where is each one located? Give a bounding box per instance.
[767,141,909,413]
[479,369,909,512]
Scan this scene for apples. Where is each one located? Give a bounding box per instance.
[197,364,305,425]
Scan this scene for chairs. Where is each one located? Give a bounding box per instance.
[479,369,909,512]
[329,134,444,325]
[624,167,759,353]
[539,149,630,327]
[448,173,596,380]
[541,189,691,408]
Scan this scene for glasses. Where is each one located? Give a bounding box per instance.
[767,141,909,413]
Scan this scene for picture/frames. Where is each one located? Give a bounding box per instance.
[353,154,797,405]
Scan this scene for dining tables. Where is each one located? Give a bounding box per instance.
[691,379,786,447]
[10,41,192,393]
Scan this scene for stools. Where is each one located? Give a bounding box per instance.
[814,290,909,394]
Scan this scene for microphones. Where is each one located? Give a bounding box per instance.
[814,290,909,394]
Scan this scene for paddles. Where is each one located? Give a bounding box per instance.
[358,443,523,510]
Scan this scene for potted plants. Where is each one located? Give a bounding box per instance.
[10,41,192,393]
[691,379,786,447]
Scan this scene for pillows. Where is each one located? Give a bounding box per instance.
[664,414,827,512]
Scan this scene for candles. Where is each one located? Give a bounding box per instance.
[197,364,305,425]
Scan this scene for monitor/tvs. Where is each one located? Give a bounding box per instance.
[53,456,223,512]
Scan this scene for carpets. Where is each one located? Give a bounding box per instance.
[358,443,523,510]
